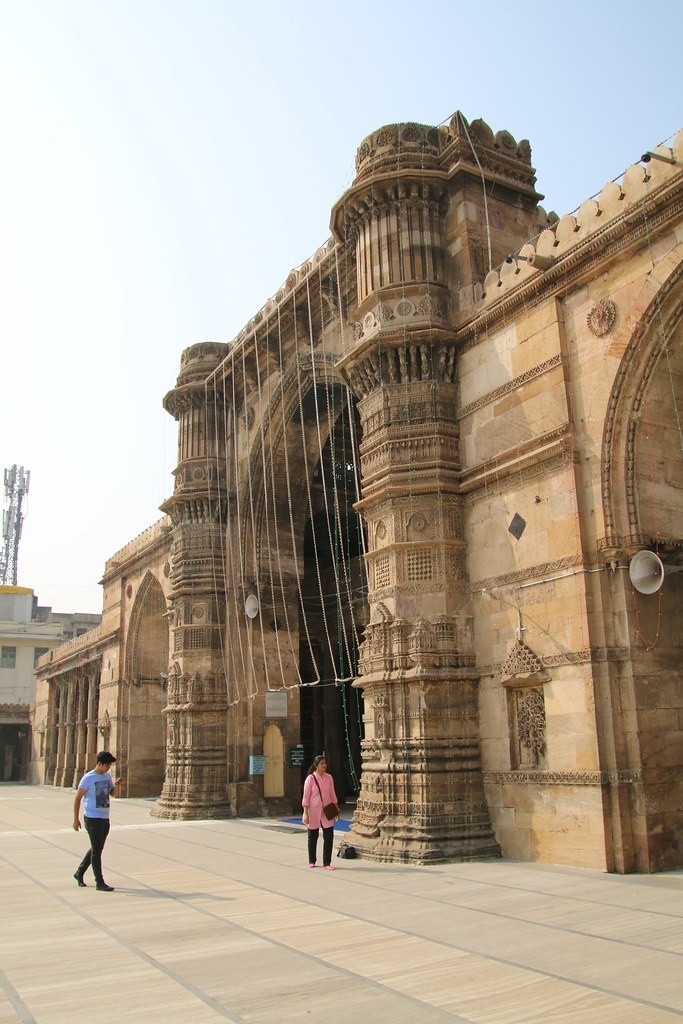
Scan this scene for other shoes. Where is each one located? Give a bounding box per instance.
[324,866,334,870]
[309,863,315,868]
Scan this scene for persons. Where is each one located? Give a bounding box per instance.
[301,756,340,870]
[72,751,124,891]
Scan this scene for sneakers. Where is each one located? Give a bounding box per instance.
[96,881,114,891]
[74,873,86,886]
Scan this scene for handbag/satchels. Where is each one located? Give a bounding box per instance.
[323,802,339,820]
[337,844,356,859]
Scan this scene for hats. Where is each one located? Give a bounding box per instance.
[97,751,116,762]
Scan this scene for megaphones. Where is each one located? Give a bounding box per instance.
[628,550,683,595]
[245,594,274,618]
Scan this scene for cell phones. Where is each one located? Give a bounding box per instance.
[115,777,123,786]
